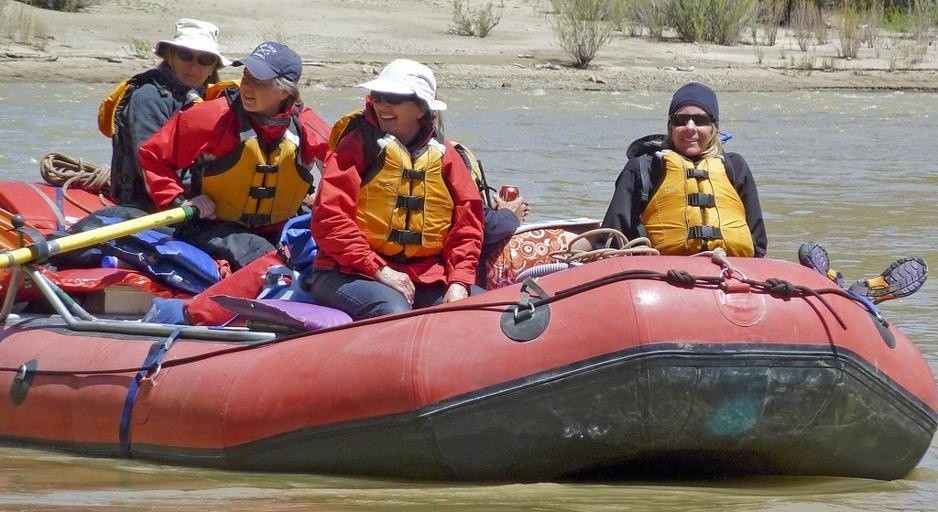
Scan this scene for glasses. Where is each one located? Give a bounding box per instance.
[368,90,416,107]
[669,111,711,127]
[170,47,217,67]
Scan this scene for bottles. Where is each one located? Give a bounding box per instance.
[498,184,516,205]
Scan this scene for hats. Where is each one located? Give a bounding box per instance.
[669,84,720,124]
[154,18,234,72]
[355,57,449,113]
[231,41,304,84]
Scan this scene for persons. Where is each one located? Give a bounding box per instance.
[596,83,928,306]
[110,18,233,214]
[137,42,332,272]
[311,60,488,321]
[477,193,532,290]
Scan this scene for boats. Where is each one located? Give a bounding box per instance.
[0,178,936,481]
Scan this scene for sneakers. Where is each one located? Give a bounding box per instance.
[798,241,844,288]
[849,257,928,305]
